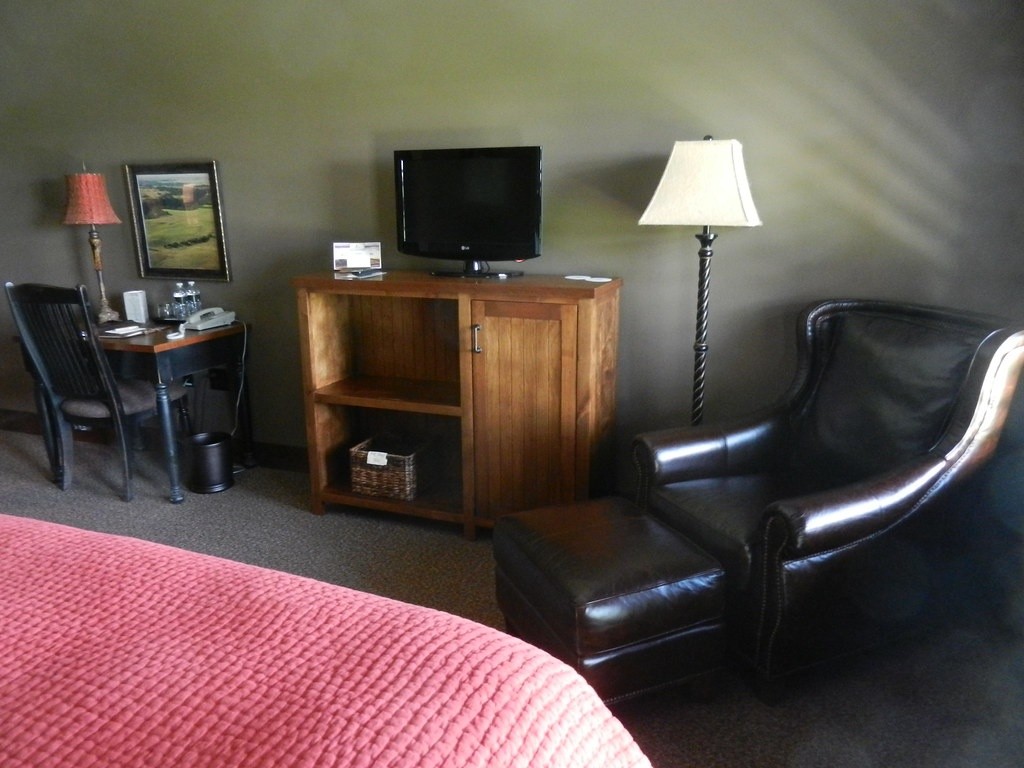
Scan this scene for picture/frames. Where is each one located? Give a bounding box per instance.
[122,160,233,283]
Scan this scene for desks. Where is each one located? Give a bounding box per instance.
[12,311,249,505]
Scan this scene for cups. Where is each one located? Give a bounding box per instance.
[157,303,172,318]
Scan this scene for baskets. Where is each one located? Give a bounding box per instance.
[350,431,426,502]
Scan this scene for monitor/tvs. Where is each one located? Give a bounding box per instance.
[393,146,544,260]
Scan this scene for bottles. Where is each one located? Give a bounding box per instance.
[185,280,202,317]
[173,282,187,322]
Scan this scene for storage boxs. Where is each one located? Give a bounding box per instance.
[350,435,439,502]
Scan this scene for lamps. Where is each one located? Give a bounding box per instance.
[636,131,765,427]
[57,165,128,323]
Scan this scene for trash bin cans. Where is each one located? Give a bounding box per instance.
[184,432,233,493]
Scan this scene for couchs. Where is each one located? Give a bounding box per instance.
[481,298,1023,717]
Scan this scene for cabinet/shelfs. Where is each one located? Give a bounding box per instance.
[292,268,628,538]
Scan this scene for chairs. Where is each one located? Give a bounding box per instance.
[5,281,195,504]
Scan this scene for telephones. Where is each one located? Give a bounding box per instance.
[180,307,235,331]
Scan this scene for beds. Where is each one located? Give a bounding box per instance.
[2,515,661,768]
